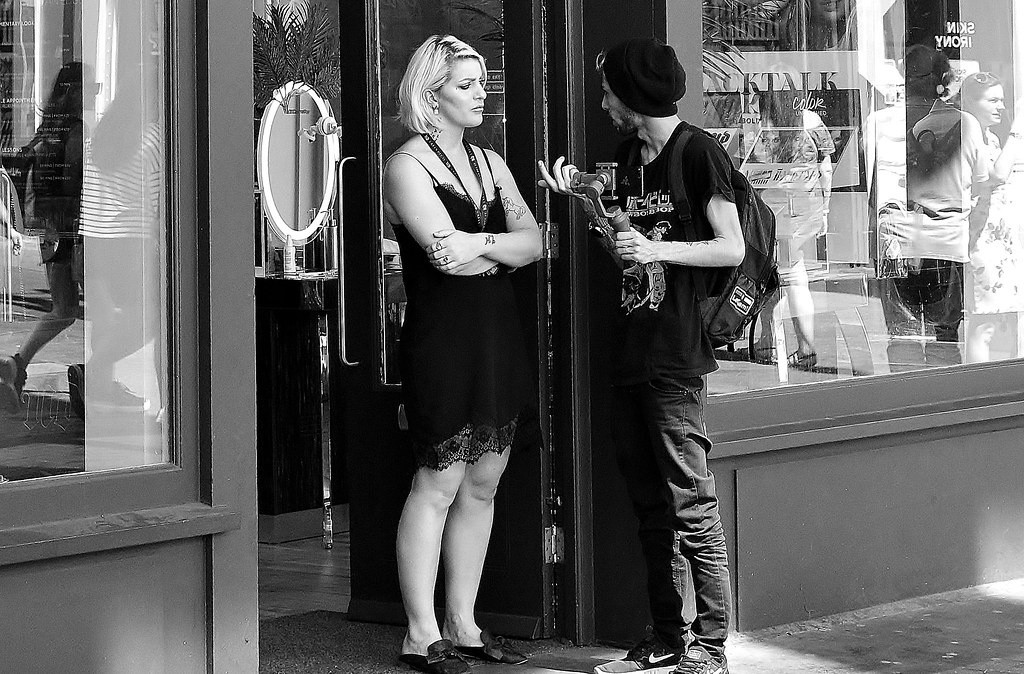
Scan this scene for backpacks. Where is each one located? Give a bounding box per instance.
[7,117,79,229]
[671,127,779,352]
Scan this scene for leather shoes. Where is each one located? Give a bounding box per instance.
[398,629,529,674]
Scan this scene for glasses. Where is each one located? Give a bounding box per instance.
[974,71,1000,82]
[595,49,608,73]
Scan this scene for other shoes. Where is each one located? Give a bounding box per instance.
[742,342,817,368]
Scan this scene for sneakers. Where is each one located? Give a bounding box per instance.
[593,638,729,674]
[67,364,87,416]
[0,354,30,413]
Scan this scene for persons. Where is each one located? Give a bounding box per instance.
[537,37,747,674]
[735,64,835,369]
[379,32,544,674]
[863,41,1023,373]
[0,59,84,423]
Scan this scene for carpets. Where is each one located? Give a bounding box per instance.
[258,610,500,674]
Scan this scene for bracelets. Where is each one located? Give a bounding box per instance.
[1007,131,1022,140]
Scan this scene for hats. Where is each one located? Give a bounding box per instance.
[604,37,687,119]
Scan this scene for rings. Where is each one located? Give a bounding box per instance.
[443,255,449,264]
[436,240,442,251]
